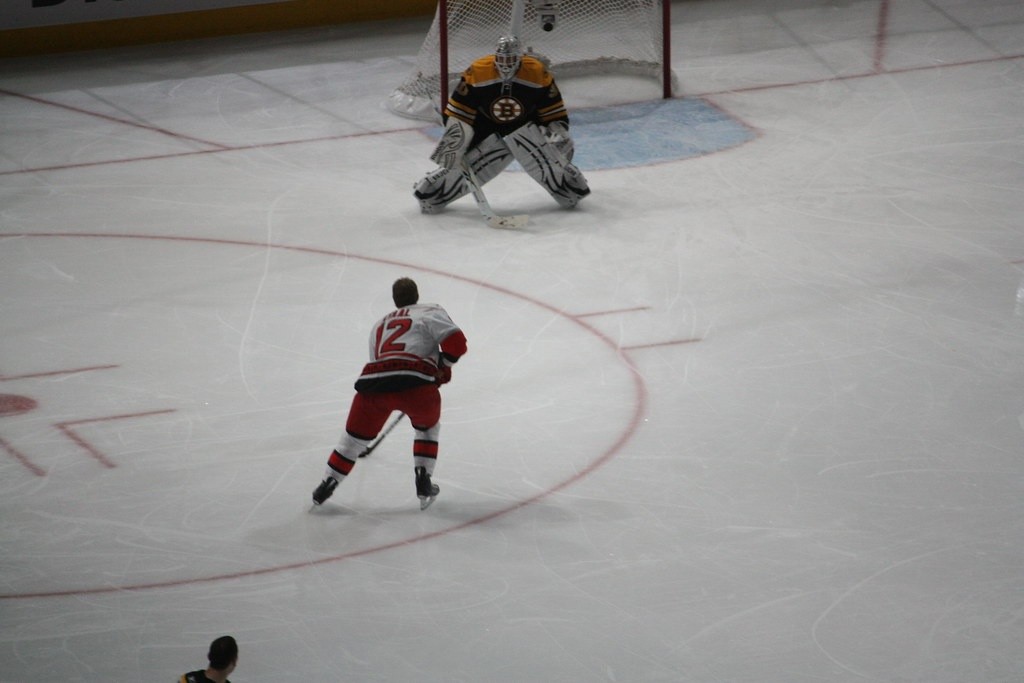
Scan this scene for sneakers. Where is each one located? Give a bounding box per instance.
[414,466,440,511]
[307,477,339,513]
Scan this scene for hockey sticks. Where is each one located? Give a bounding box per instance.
[354,376,438,456]
[460,151,529,231]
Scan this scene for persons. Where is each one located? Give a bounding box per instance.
[177,636,238,683]
[311,277,472,512]
[414,34,591,215]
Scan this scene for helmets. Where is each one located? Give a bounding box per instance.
[497,37,522,54]
